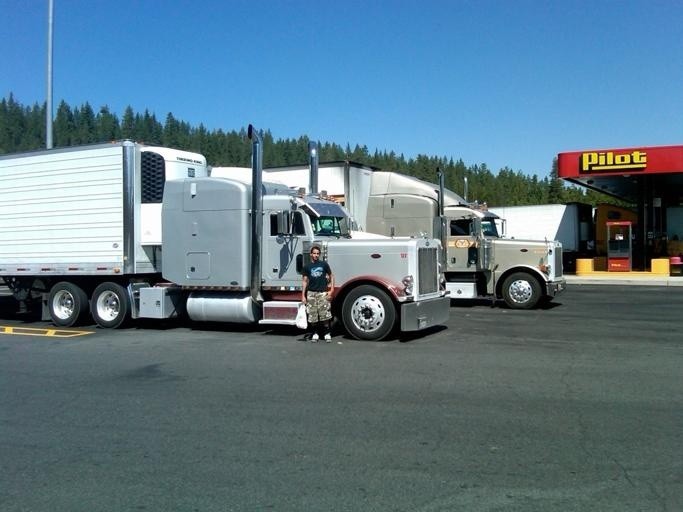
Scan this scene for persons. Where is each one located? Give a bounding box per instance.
[587,235,595,258]
[301,247,334,343]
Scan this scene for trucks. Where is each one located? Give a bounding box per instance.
[0,139,451,341]
[208,157,565,311]
[485,202,637,269]
